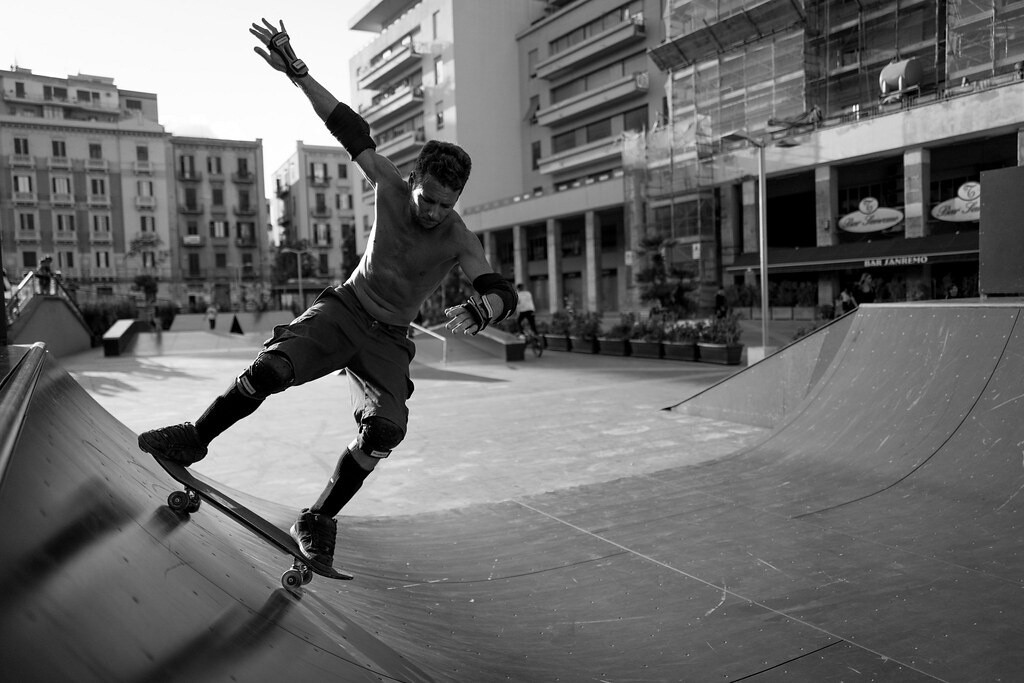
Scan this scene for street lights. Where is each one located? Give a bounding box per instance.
[282,248,311,313]
[722,131,770,346]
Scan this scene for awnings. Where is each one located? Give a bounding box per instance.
[727,231,980,274]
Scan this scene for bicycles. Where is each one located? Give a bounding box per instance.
[506,314,544,358]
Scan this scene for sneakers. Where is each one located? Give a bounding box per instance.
[290,508,337,568]
[137,421,208,468]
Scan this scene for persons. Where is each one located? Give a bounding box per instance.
[201,302,218,330]
[517,283,539,340]
[39,257,52,295]
[138,17,523,568]
[841,272,964,312]
[714,286,728,321]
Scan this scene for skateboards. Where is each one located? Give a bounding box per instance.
[152,450,355,590]
